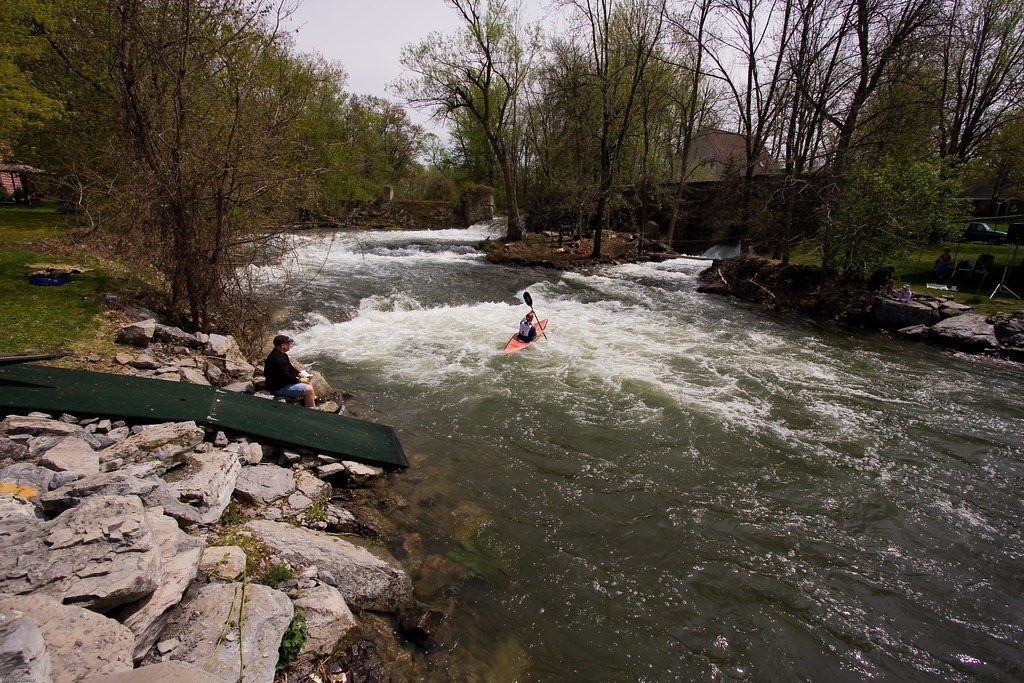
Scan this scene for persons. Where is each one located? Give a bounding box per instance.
[867,266,897,306]
[899,284,912,302]
[264,335,315,406]
[518,310,537,342]
[934,248,951,274]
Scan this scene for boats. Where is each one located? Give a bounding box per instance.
[501,319,549,354]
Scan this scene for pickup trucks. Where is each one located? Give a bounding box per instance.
[942,221,1007,245]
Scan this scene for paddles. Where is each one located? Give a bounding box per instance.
[523,292,547,339]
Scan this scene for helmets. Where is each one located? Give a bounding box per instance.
[527,313,533,319]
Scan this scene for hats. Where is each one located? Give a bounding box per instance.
[273,335,291,345]
[903,284,910,289]
